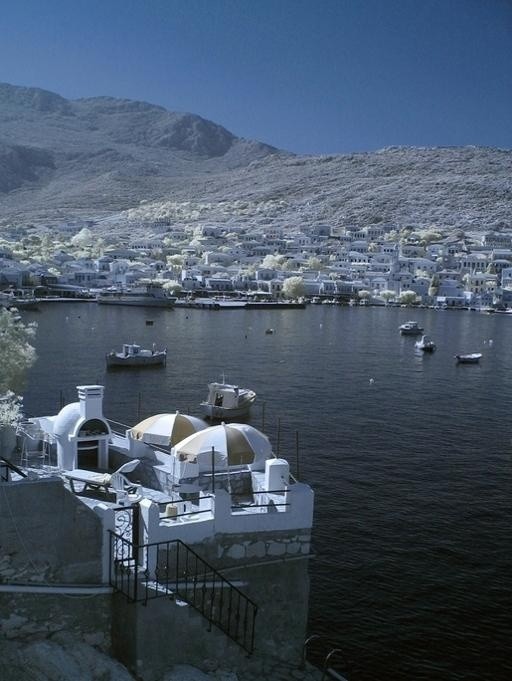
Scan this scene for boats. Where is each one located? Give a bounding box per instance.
[199,370,256,418]
[397,320,436,350]
[297,296,369,306]
[106,341,169,367]
[455,353,483,362]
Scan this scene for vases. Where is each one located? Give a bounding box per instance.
[0,421,39,463]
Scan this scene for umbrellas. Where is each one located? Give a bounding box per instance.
[171,420,272,492]
[129,409,211,506]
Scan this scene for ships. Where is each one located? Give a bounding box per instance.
[96,279,176,309]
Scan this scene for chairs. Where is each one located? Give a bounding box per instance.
[110,471,143,512]
[16,428,51,468]
[62,459,140,500]
